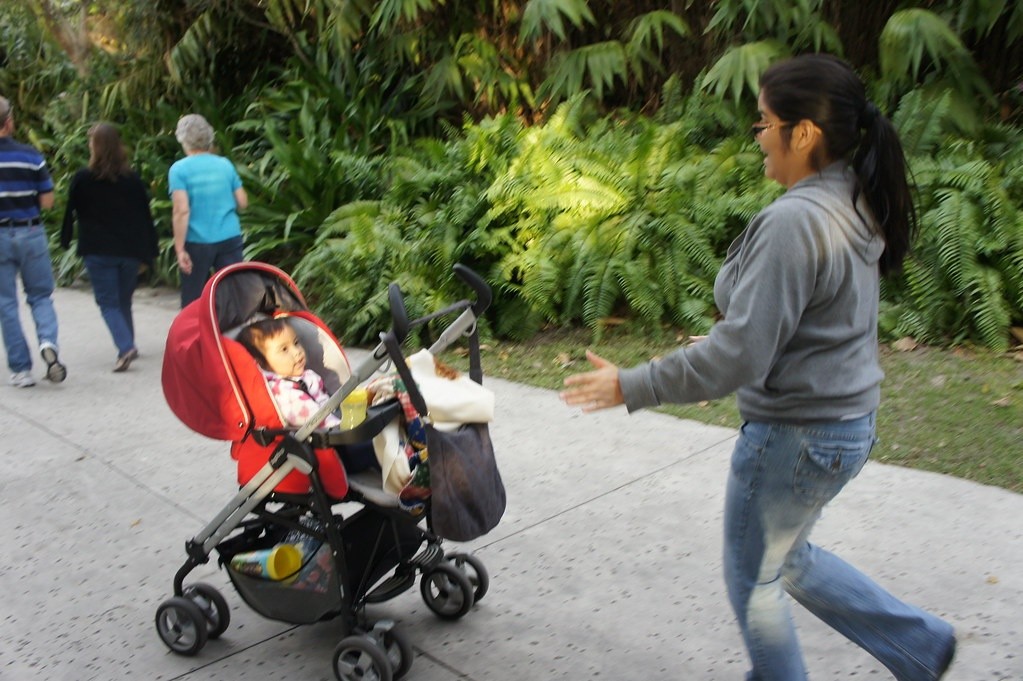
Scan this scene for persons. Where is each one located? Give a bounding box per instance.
[0,96,67,387]
[169,114,247,308]
[61,122,160,371]
[234,317,380,474]
[558,55,960,680]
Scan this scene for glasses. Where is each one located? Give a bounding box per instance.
[750,120,792,133]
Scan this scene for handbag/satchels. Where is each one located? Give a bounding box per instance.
[423,423,506,540]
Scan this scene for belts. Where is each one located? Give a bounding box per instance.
[0,216,41,228]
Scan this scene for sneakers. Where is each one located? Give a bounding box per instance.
[39,341,66,383]
[7,370,36,388]
[112,347,136,372]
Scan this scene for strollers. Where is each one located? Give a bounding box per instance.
[152,262,493,680]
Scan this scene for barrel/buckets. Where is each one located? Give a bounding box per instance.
[229,543,302,586]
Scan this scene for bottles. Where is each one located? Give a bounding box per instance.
[339,389,368,430]
